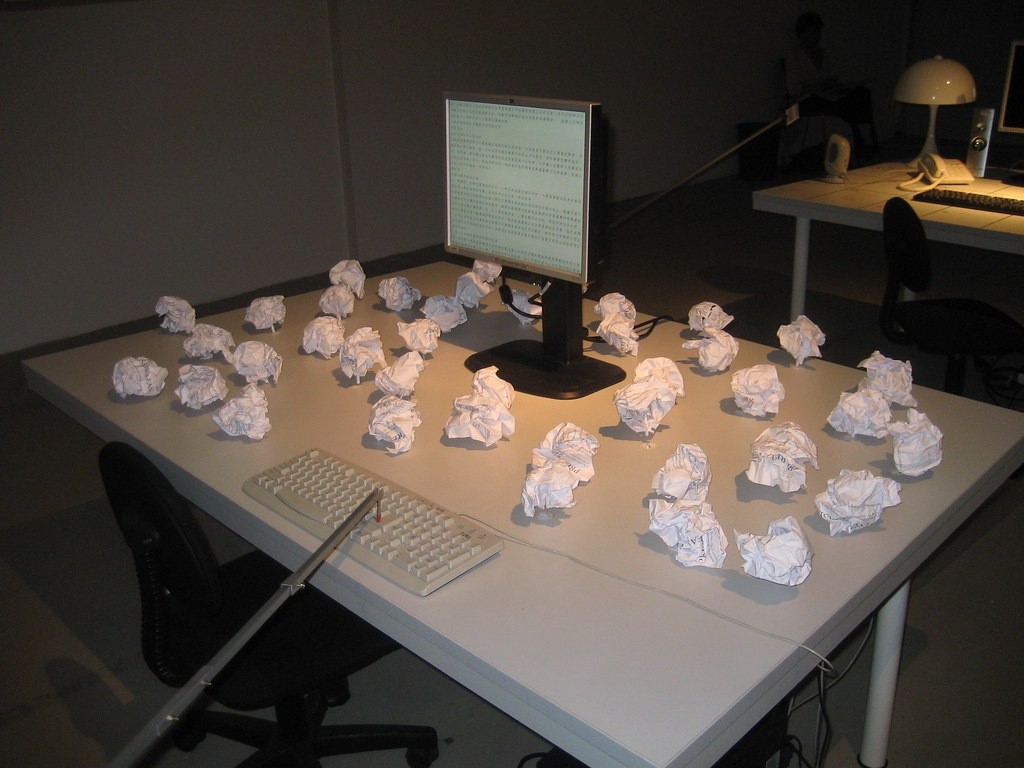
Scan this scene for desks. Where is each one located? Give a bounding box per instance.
[751,162,1024,325]
[20,261,1024,768]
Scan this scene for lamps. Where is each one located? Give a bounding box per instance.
[893,56,976,168]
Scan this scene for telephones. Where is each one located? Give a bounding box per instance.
[917,153,975,185]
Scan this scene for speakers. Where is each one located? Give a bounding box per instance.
[818,133,851,183]
[966,108,994,177]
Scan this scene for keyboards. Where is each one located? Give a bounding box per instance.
[913,189,1024,216]
[242,448,504,597]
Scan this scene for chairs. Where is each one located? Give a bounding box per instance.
[880,197,1024,396]
[777,57,835,166]
[98,440,438,768]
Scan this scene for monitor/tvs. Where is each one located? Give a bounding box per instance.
[998,40,1024,133]
[441,91,627,400]
